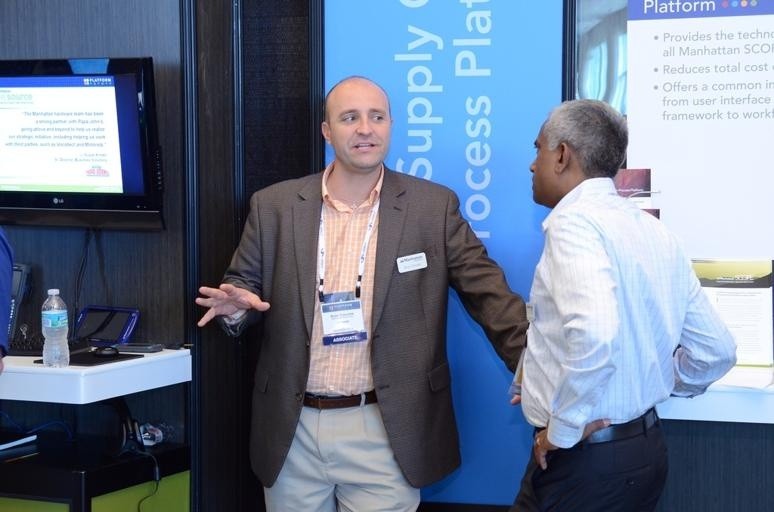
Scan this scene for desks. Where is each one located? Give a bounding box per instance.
[0,345,192,512]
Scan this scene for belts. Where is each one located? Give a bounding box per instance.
[587,411,658,444]
[303,392,377,409]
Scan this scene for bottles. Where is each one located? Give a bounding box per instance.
[39,288,70,367]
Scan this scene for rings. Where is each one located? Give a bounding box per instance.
[536,438,540,446]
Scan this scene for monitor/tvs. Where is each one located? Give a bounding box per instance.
[73,305,139,346]
[0,56,165,233]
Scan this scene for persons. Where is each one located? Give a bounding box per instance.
[510,97,739,511]
[194,74,529,511]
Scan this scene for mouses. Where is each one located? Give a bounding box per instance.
[96,346,117,358]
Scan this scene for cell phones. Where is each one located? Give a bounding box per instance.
[118,342,163,353]
